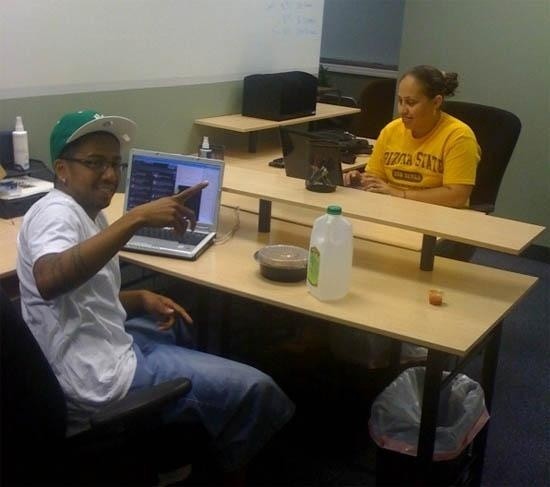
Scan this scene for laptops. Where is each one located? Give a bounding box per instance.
[278,125,345,187]
[121,147,226,261]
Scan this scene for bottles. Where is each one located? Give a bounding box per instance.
[198,135,214,160]
[11,115,31,171]
[304,202,357,305]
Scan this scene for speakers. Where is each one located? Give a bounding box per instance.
[241,70,319,122]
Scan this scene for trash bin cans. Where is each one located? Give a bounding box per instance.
[369,367,489,487]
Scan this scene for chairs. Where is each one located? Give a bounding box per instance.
[438,96,522,261]
[2,280,188,486]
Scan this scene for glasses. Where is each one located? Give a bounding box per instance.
[59,156,130,173]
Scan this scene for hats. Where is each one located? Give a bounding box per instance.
[50,106,138,165]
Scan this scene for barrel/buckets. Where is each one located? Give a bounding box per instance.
[307,205,354,302]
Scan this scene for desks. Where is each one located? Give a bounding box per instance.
[192,103,375,180]
[118,163,547,485]
[0,163,124,279]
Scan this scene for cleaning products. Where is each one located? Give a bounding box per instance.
[12,116,29,169]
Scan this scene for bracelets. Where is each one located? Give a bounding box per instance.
[402,188,406,198]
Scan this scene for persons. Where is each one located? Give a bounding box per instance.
[15,107,298,484]
[280,62,484,354]
